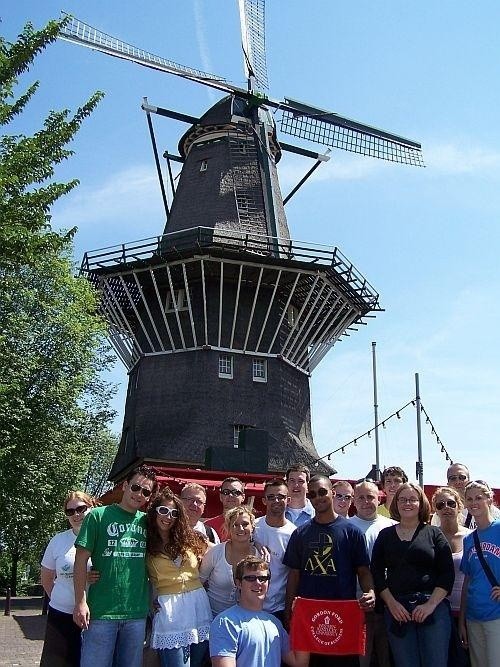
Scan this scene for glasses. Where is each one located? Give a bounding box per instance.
[306,488,333,499]
[435,500,458,510]
[181,497,207,506]
[448,475,469,482]
[464,480,487,488]
[127,483,152,497]
[64,505,88,517]
[155,505,179,519]
[397,497,420,503]
[240,574,271,582]
[335,494,352,500]
[265,494,287,500]
[219,489,243,496]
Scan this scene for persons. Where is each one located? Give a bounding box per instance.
[431,487,472,665]
[376,465,408,517]
[152,507,270,630]
[209,556,310,667]
[460,480,500,665]
[432,464,498,524]
[283,463,314,528]
[207,476,245,541]
[331,480,353,519]
[181,484,220,544]
[40,491,94,665]
[282,474,376,667]
[73,465,159,665]
[87,491,207,666]
[349,478,400,667]
[371,483,454,667]
[254,480,295,621]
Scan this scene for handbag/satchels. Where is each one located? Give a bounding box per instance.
[373,578,391,615]
